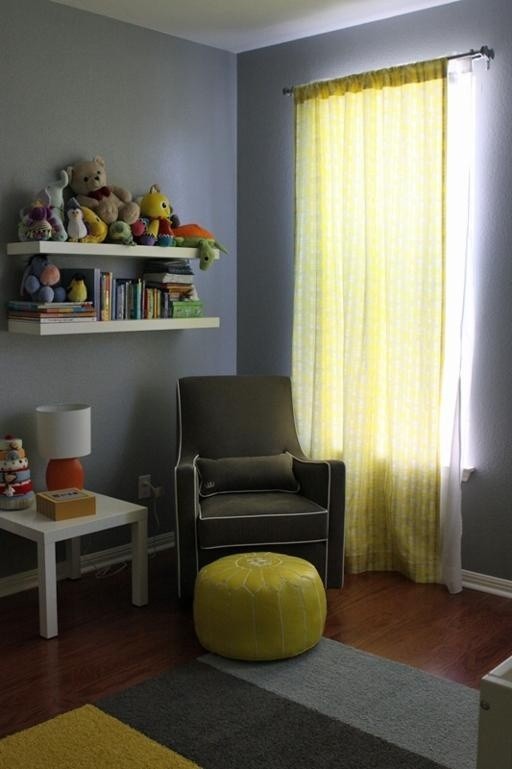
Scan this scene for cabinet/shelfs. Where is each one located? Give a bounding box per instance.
[7,241,221,336]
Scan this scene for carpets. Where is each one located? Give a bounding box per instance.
[0,635,479,768]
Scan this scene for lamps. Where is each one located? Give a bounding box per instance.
[36,403,92,491]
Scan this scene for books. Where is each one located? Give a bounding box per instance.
[11,258,205,324]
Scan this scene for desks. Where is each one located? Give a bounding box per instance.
[1,489,149,640]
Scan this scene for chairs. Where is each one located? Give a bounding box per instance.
[174,374,347,598]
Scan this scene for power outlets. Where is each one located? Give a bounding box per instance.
[138,474,151,500]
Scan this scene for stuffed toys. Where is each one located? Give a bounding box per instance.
[20,253,67,302]
[18,156,229,271]
